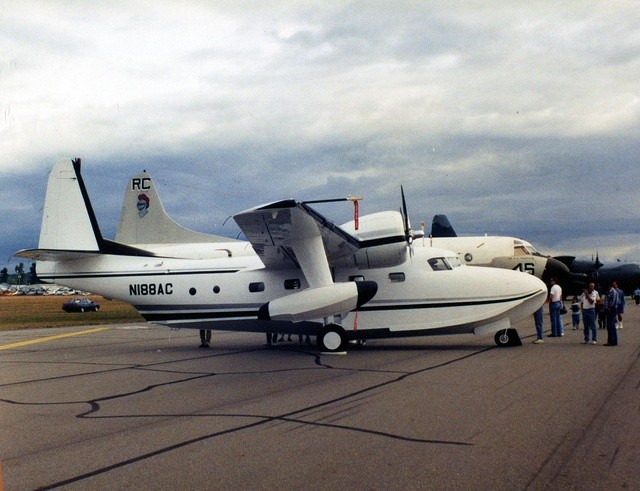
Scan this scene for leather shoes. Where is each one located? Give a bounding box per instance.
[558,334,562,337]
[547,334,557,338]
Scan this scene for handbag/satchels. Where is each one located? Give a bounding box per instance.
[560,303,567,315]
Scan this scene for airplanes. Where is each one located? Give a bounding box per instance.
[111,173,569,321]
[429,215,640,303]
[11,156,549,354]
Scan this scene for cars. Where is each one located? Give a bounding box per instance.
[61,298,99,313]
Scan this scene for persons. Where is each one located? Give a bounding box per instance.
[569,296,581,330]
[612,280,625,329]
[603,281,619,346]
[581,283,597,344]
[547,277,563,337]
[634,288,640,305]
[589,282,600,330]
[532,306,544,344]
[598,294,606,329]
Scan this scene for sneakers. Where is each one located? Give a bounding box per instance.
[619,323,623,329]
[603,342,615,346]
[615,324,619,330]
[584,340,589,344]
[533,340,544,344]
[591,341,597,345]
